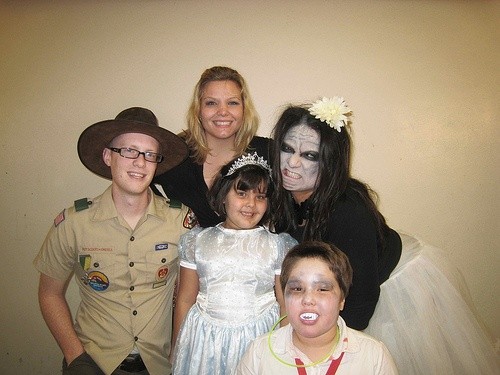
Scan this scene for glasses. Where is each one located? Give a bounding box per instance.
[108,147,162,163]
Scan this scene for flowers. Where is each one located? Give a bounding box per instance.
[308,95,351,132]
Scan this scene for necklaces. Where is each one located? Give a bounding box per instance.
[269,314,340,368]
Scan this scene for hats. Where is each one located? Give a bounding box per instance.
[77,107,190,179]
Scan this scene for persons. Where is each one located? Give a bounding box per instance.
[260,97,402,332]
[232,241,397,374]
[153,66,273,229]
[171,151,299,374]
[32,107,200,375]
